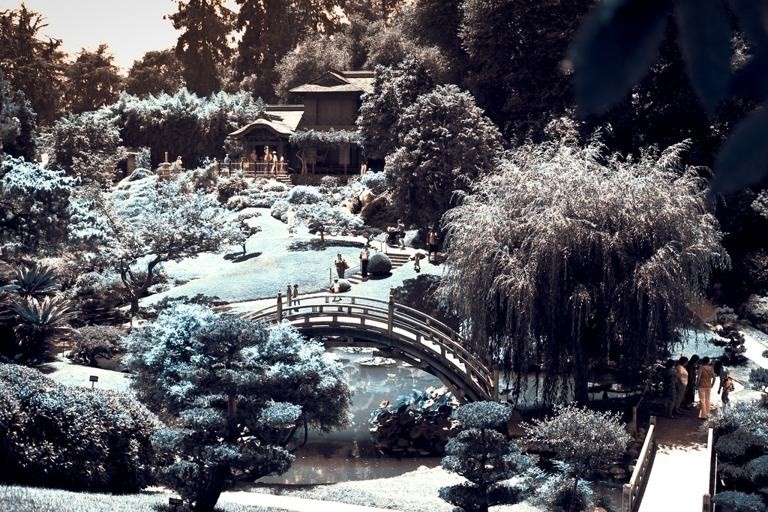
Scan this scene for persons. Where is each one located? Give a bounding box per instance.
[414,252,421,270]
[293,284,300,305]
[334,253,349,278]
[202,153,232,168]
[248,146,286,173]
[331,278,342,302]
[359,243,370,282]
[658,355,734,422]
[426,223,441,264]
[395,219,406,249]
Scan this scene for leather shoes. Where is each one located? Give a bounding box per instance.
[667,404,697,419]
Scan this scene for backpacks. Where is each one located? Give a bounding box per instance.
[724,378,735,393]
